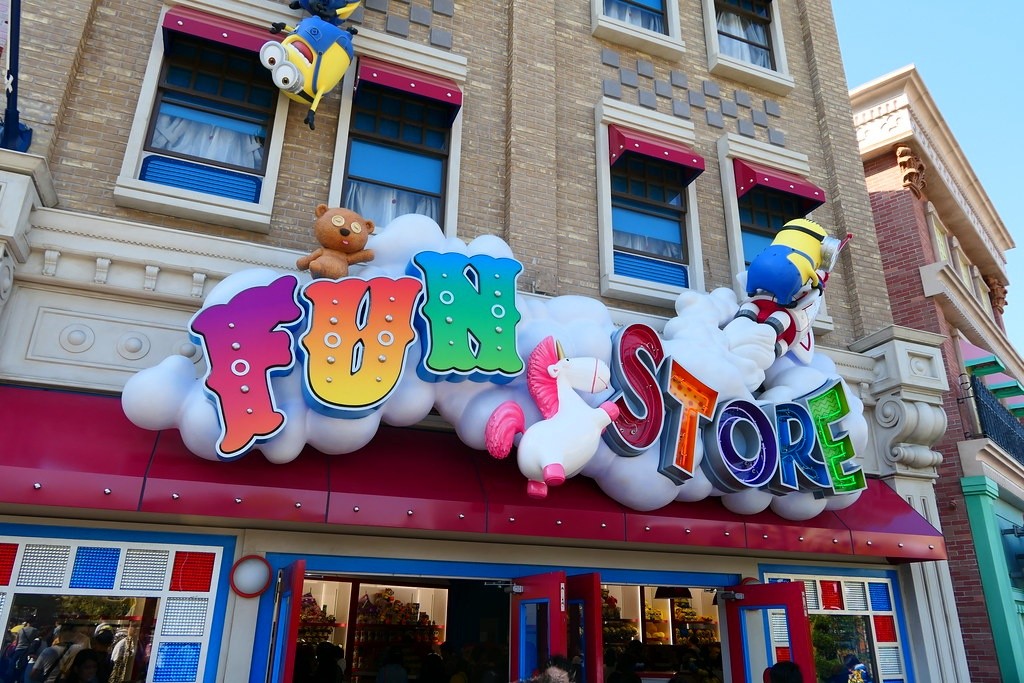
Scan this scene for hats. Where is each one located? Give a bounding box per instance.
[93,623,115,638]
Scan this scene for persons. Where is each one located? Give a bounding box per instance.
[763,660,804,683]
[0,614,116,683]
[844,654,872,683]
[530,634,726,683]
[293,634,508,683]
